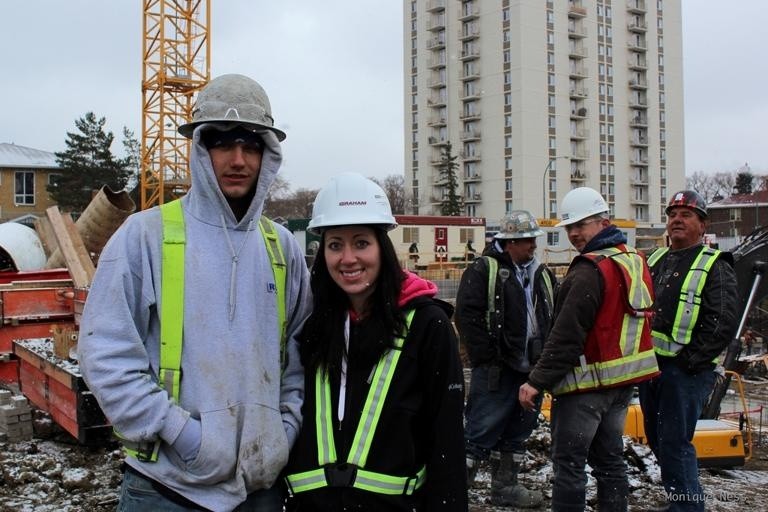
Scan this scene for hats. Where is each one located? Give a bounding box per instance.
[177,74,287,143]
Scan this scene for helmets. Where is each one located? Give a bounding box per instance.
[493,210,544,240]
[665,190,708,219]
[553,187,609,228]
[306,177,400,236]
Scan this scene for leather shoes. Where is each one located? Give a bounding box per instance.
[490,482,543,508]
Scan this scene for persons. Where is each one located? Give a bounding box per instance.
[520,186,663,512]
[76,74,312,512]
[267,173,469,512]
[462,240,476,261]
[455,210,560,508]
[638,190,739,512]
[409,241,419,263]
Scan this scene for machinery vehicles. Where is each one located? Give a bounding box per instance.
[537,228,768,470]
